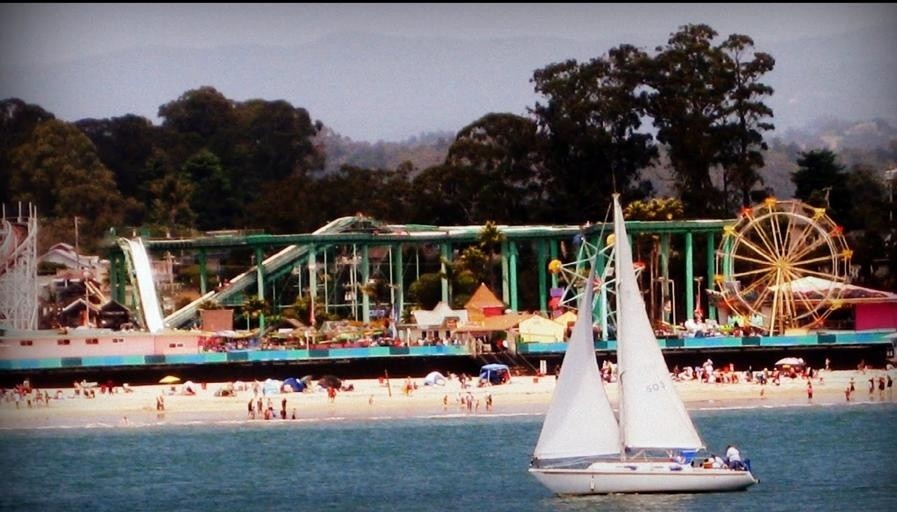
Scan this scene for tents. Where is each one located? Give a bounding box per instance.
[480,364,512,385]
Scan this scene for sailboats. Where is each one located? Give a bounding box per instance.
[529,194,758,496]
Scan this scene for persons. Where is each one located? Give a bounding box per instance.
[156,382,207,410]
[691,445,741,470]
[3,381,132,409]
[673,359,895,402]
[226,370,492,419]
[215,334,506,354]
[602,360,613,383]
[732,315,761,336]
